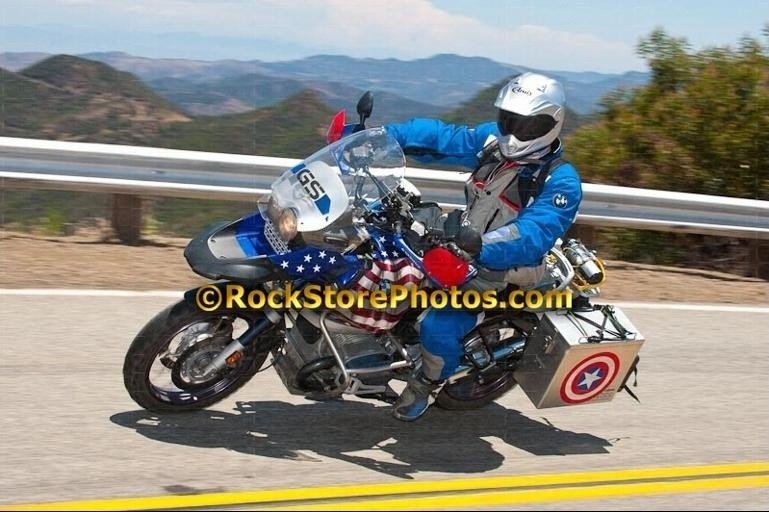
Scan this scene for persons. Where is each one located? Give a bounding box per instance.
[327,64,584,423]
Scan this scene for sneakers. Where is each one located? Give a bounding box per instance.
[391,369,448,422]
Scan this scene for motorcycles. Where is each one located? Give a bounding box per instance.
[123,91,644,414]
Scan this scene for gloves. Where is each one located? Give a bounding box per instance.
[341,125,393,169]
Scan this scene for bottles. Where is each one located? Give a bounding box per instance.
[561,238,603,285]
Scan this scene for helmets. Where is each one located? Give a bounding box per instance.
[494,71,566,159]
